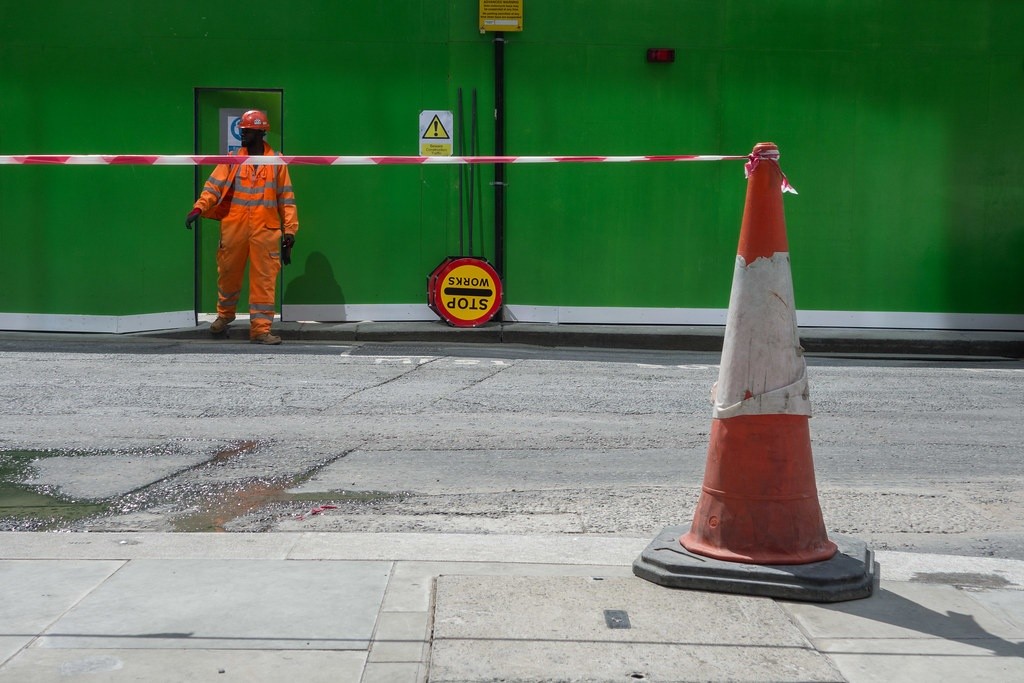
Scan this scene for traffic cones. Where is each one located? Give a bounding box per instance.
[632,141,880,604]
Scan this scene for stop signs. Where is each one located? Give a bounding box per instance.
[433,259,503,327]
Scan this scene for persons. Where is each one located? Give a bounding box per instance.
[184,109,298,344]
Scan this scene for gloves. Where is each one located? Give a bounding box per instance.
[185,207,202,230]
[283,234,295,249]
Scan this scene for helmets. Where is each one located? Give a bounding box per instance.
[235,109,270,132]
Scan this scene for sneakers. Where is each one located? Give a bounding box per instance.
[209,316,236,333]
[249,331,282,345]
